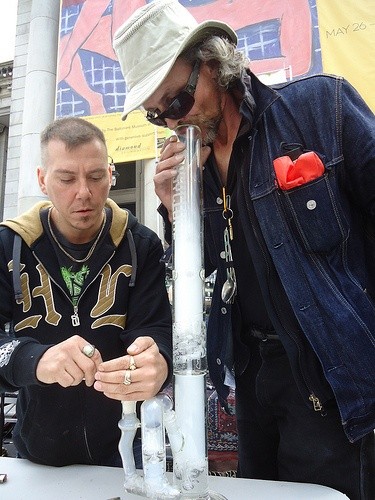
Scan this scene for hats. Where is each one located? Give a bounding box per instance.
[113,0,237,121]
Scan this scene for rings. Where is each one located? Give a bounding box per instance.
[123,370,131,386]
[129,356,136,371]
[82,344,95,357]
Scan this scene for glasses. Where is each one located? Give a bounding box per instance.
[146,57,201,128]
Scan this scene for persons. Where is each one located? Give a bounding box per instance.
[114,0,375,500]
[0,117,173,470]
